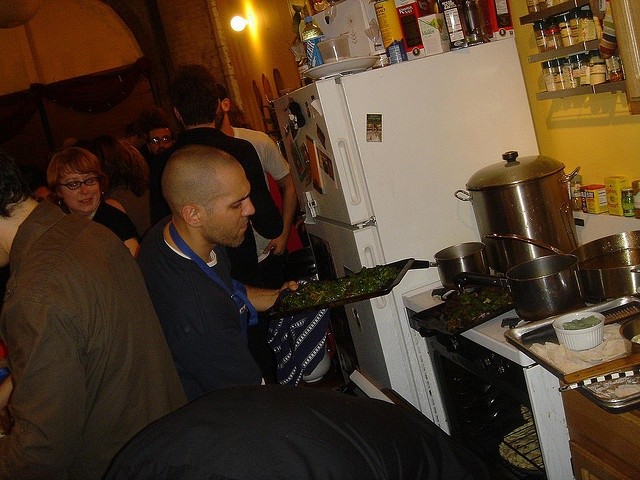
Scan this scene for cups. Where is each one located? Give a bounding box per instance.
[316,35,352,63]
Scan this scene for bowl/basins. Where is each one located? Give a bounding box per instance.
[552,310,605,351]
[619,317,640,357]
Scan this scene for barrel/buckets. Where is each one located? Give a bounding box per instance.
[454,150,580,271]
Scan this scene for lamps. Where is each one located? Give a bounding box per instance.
[230,15,254,32]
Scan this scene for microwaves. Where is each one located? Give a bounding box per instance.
[313,1,386,69]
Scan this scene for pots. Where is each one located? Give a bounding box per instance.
[483,230,640,302]
[408,242,490,290]
[454,151,581,274]
[454,255,585,321]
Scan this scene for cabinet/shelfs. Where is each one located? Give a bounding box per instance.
[609,0,640,115]
[519,0,626,101]
[560,385,639,479]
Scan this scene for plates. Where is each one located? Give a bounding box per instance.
[304,55,379,80]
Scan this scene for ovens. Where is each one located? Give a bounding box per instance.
[405,308,573,480]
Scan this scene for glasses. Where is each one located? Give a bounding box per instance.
[149,136,172,145]
[57,176,100,191]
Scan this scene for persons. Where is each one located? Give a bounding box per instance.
[102,385,487,480]
[133,144,300,402]
[215,83,298,289]
[124,120,140,148]
[0,148,188,478]
[45,147,142,261]
[147,63,284,384]
[85,136,153,238]
[30,145,62,201]
[143,119,177,158]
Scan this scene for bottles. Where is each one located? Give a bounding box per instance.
[621,188,634,217]
[606,56,622,82]
[568,52,587,87]
[542,61,557,91]
[570,176,583,211]
[301,16,325,68]
[552,57,565,90]
[590,49,607,87]
[578,54,590,86]
[578,10,595,42]
[526,0,538,13]
[544,16,564,50]
[532,19,548,53]
[559,14,573,48]
[632,180,640,218]
[568,6,581,45]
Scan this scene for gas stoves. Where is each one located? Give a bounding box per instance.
[403,275,531,353]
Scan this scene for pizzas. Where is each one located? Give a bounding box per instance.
[498,422,543,469]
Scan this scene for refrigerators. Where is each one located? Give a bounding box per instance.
[271,42,541,435]
